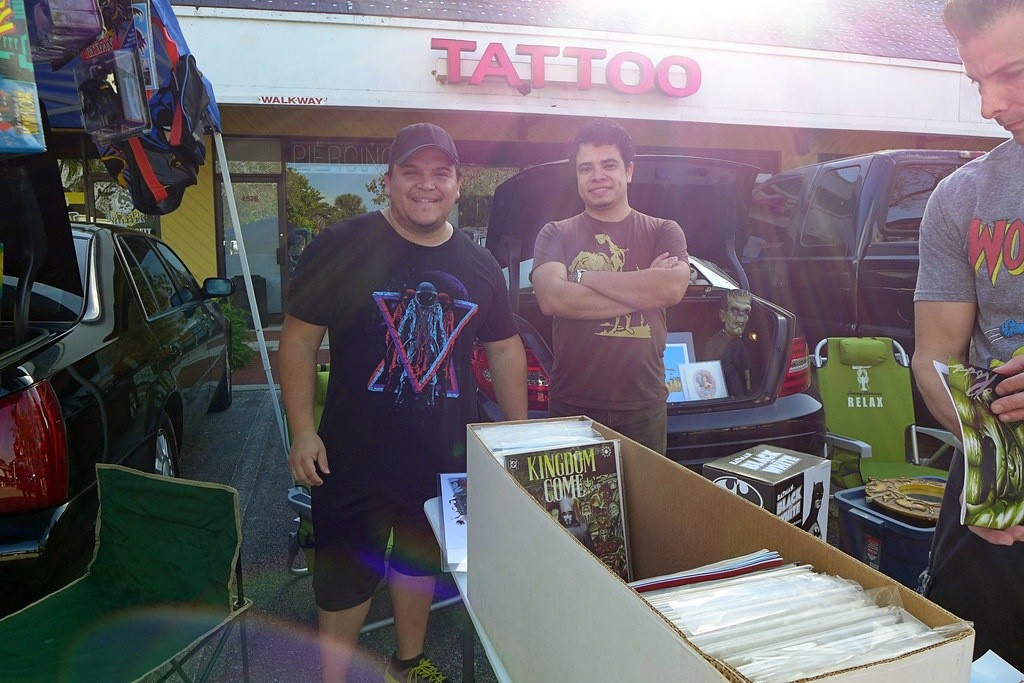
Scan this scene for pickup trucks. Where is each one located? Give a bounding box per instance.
[756,150,995,346]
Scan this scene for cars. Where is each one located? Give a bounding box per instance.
[0,120,236,589]
[461,154,824,472]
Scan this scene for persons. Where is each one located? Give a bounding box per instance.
[529,118,689,457]
[704,289,755,397]
[913,0,1024,673]
[278,122,528,683]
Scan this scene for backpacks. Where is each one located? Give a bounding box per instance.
[117,52,211,217]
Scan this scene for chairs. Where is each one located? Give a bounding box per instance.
[815,337,959,520]
[0,463,253,683]
[287,364,462,633]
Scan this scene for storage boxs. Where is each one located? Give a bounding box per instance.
[703,444,831,543]
[0,0,153,160]
[467,414,975,683]
[834,476,948,596]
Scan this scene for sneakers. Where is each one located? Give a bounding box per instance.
[383,648,454,683]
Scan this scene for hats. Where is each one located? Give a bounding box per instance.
[387,122,459,167]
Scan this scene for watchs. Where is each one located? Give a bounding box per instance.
[569,269,586,284]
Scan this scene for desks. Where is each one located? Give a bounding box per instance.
[424,497,512,682]
